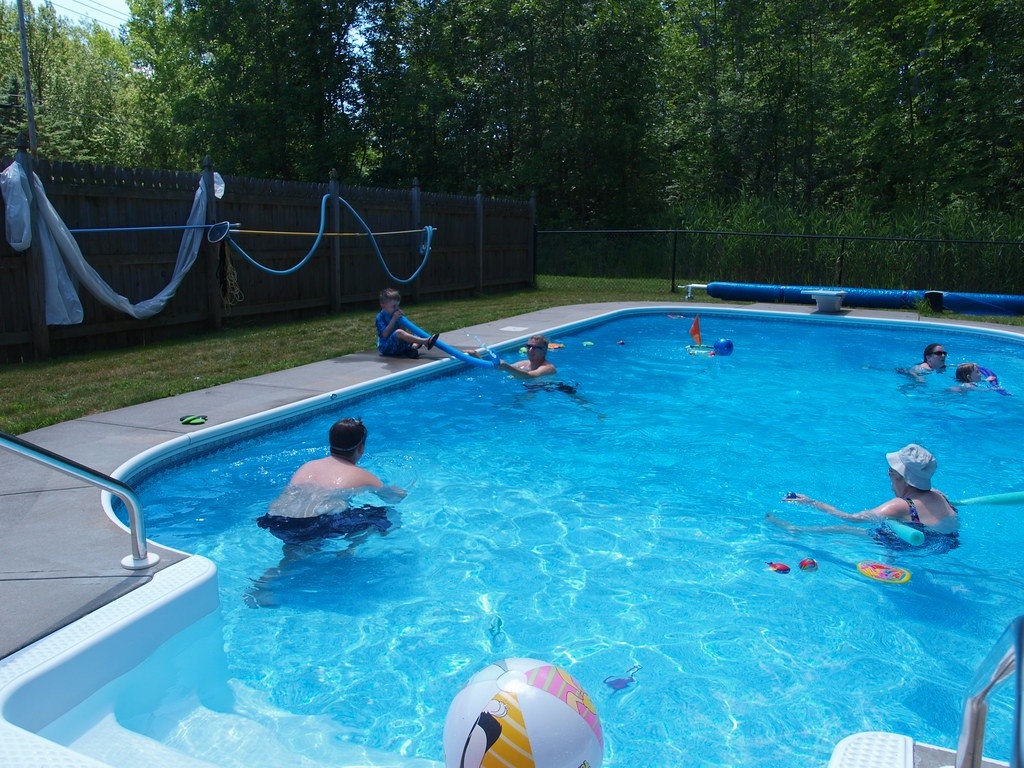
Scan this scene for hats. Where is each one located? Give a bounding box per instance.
[886,442,937,491]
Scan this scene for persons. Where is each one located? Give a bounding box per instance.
[955,363,996,383]
[462,335,557,377]
[375,288,440,359]
[781,445,956,527]
[919,343,948,369]
[256,419,407,529]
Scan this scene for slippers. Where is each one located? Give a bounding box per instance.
[427,333,440,351]
[404,347,418,358]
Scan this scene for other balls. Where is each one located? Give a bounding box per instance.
[443,658,604,768]
[714,338,733,356]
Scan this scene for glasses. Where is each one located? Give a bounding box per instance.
[931,351,947,356]
[525,344,542,350]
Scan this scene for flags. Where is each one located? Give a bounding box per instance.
[688,316,702,347]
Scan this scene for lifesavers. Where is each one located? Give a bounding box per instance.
[883,490,1024,546]
[975,366,1012,397]
